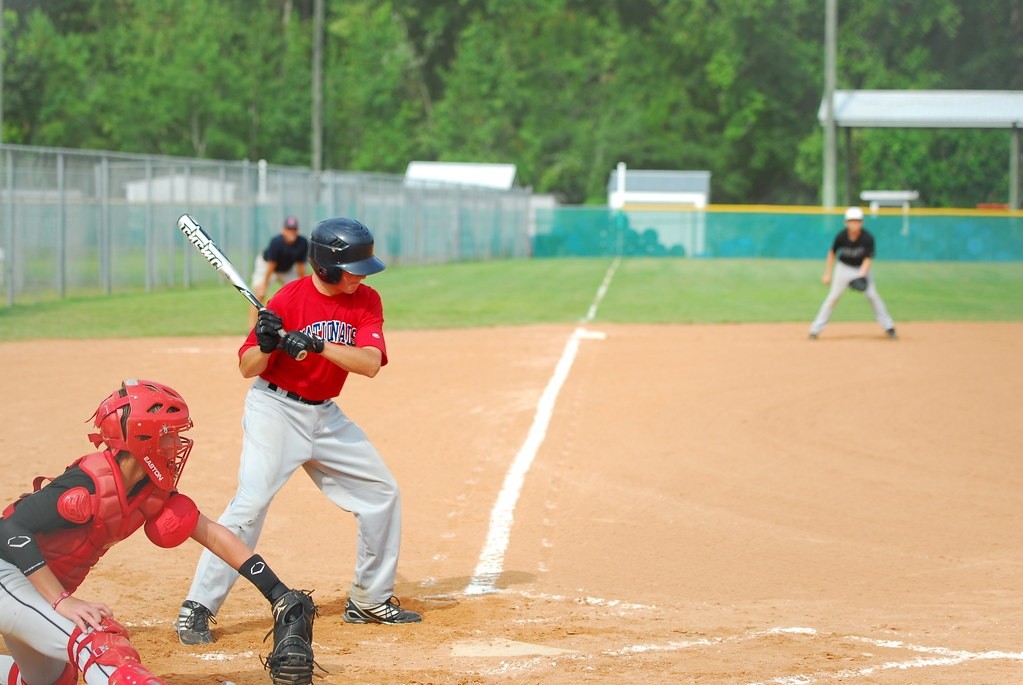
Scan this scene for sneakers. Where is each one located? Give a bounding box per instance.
[175,600,213,645]
[342,598,423,624]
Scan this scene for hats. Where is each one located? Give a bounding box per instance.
[845,206,863,221]
[284,216,298,229]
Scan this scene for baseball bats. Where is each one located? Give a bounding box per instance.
[175,213,307,362]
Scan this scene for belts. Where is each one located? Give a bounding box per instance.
[269,382,330,406]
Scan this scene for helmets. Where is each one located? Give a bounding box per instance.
[307,217,386,284]
[99,380,194,491]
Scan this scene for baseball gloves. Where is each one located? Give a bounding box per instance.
[257,588,329,685]
[849,277,868,292]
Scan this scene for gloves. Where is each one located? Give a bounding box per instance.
[277,330,325,359]
[255,310,283,353]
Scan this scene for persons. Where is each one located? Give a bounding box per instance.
[808,207,897,339]
[247,219,309,340]
[0,380,313,685]
[175,219,421,648]
